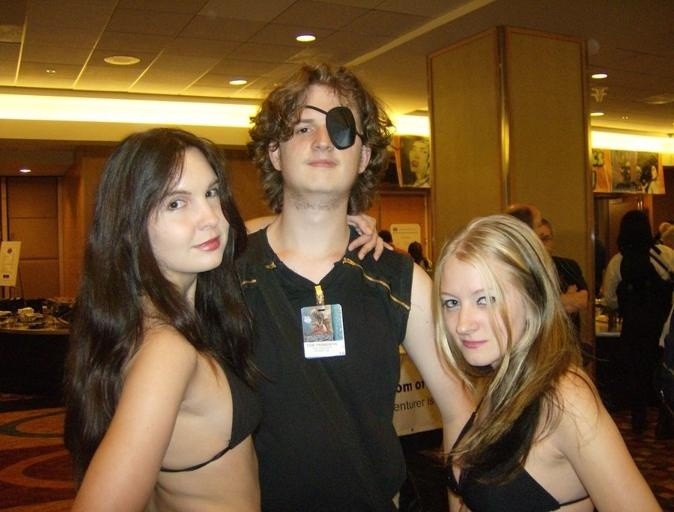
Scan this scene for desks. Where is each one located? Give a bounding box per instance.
[2,296,80,396]
[595,296,632,410]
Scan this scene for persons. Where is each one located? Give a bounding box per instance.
[196,59,475,511]
[613,160,659,194]
[60,129,397,512]
[371,203,674,437]
[432,215,664,510]
[408,137,431,188]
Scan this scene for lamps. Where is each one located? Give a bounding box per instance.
[382,112,429,139]
[585,124,674,159]
[0,84,265,135]
